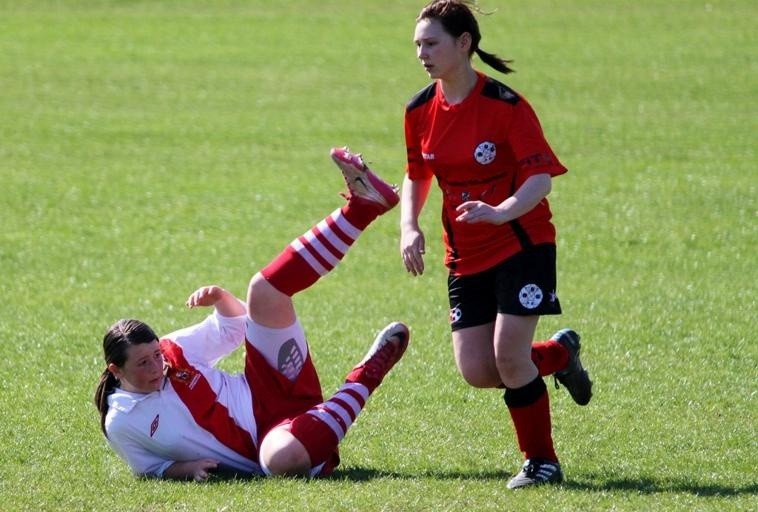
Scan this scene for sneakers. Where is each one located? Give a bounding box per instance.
[329,145,402,213]
[548,327,592,407]
[352,321,411,388]
[505,455,566,490]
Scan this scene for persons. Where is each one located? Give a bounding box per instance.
[398,1,593,489]
[93,146,409,485]
